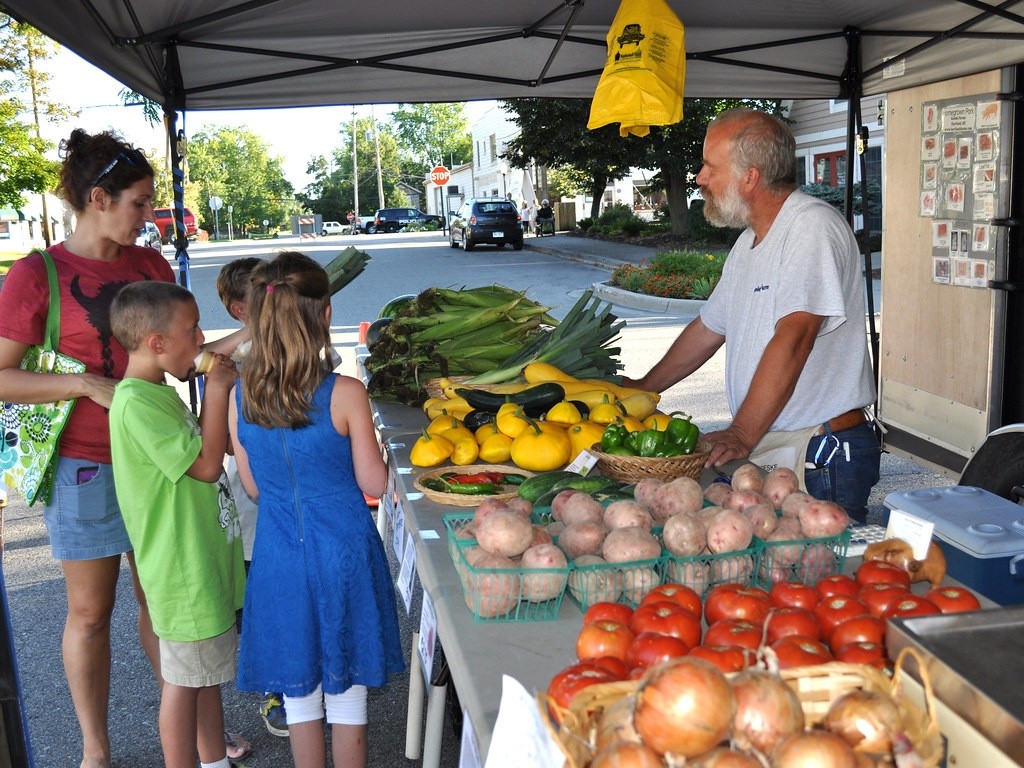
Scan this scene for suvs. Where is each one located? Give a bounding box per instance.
[317,221,354,237]
[448,195,524,252]
[373,207,447,233]
[151,206,200,246]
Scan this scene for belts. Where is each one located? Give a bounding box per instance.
[811,408,865,437]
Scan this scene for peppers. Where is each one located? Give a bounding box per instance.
[434,414,701,497]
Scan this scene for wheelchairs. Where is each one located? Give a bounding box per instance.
[535,212,556,238]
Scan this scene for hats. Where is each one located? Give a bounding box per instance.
[540,199,549,205]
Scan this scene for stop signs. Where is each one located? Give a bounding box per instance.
[430,166,451,186]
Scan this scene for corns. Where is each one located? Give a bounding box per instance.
[363,285,561,403]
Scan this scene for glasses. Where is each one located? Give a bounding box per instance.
[88,149,141,202]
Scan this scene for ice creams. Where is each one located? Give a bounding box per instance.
[193,348,217,373]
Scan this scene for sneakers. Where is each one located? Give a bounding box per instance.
[260,693,289,736]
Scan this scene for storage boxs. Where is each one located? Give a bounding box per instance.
[880,484,1024,609]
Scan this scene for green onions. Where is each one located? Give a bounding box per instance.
[453,290,627,387]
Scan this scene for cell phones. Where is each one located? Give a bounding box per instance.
[77,467,99,485]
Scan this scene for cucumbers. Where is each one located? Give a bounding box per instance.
[518,471,584,501]
[552,476,618,494]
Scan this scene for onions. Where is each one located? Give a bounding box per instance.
[585,658,902,768]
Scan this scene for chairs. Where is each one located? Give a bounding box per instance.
[957,422,1024,505]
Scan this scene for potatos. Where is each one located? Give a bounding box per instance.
[454,463,850,618]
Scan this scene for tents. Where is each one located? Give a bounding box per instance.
[1,0,1024,423]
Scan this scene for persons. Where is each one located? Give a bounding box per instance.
[110,281,242,767]
[536,199,554,225]
[507,193,517,208]
[218,258,292,738]
[624,109,880,527]
[530,199,540,234]
[519,200,530,233]
[0,131,255,768]
[228,253,406,767]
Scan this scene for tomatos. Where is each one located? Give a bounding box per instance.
[546,557,980,728]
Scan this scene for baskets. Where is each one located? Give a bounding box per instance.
[590,440,711,487]
[441,496,852,623]
[412,463,537,507]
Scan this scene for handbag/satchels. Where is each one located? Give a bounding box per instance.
[0,251,88,507]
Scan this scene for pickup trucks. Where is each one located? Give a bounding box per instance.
[355,214,398,234]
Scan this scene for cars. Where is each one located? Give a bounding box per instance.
[134,222,163,256]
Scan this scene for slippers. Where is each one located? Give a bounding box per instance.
[223,732,252,761]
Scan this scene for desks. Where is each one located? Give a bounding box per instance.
[354,343,1002,768]
